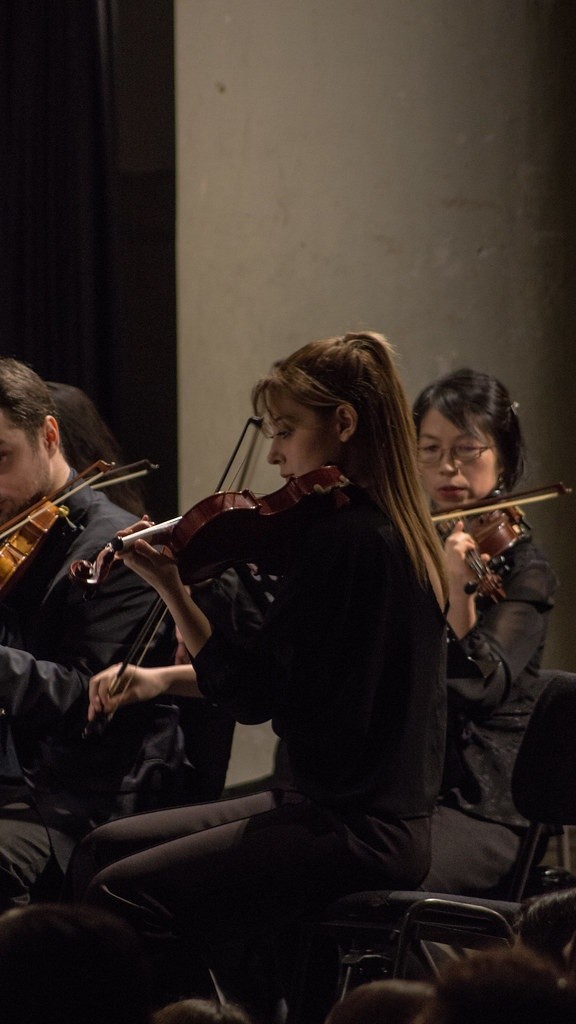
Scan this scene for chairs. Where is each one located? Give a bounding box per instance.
[313,674,575,987]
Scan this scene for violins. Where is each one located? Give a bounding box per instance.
[436,496,531,607]
[0,495,68,606]
[65,466,357,587]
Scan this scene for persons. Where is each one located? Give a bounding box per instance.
[40,331,453,1023]
[411,370,556,896]
[1,355,176,914]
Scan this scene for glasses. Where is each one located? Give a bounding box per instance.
[415,444,496,464]
